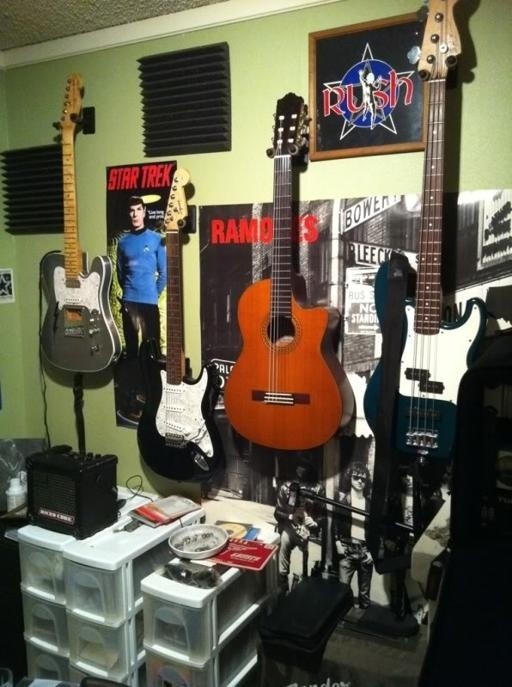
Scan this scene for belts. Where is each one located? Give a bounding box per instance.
[340,538,369,551]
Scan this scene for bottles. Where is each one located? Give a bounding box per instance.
[5,478,26,512]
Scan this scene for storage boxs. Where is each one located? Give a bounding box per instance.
[140,516,278,687]
[17,486,205,687]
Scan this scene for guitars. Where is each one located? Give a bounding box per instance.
[224,92,356,450]
[362,0,486,468]
[137,168,226,483]
[39,74,123,374]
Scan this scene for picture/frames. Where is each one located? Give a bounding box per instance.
[307,9,437,159]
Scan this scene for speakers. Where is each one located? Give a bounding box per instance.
[24,444,118,539]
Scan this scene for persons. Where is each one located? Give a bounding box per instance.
[274,460,327,593]
[115,195,168,360]
[331,461,375,610]
[359,68,382,129]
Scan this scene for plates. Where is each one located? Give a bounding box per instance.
[169,524,230,560]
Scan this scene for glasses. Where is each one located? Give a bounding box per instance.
[349,472,368,482]
